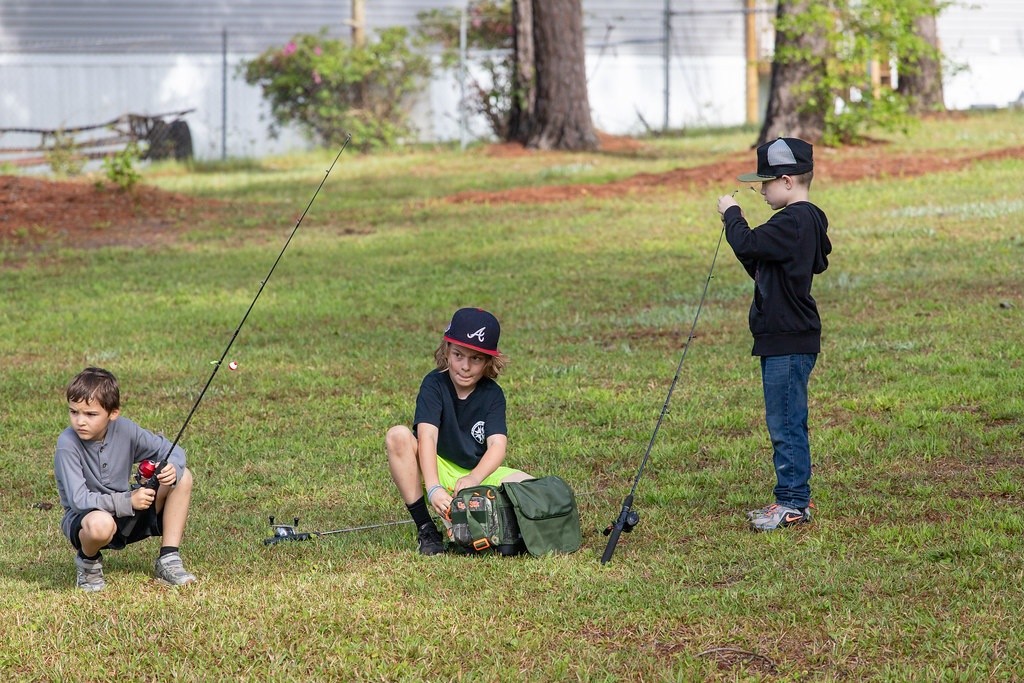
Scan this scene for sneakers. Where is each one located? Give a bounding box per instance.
[417,523,444,555]
[749,505,813,531]
[747,503,778,521]
[153,551,198,586]
[75,549,106,592]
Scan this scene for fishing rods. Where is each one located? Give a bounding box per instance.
[600,191,740,561]
[262,515,441,548]
[123,131,352,538]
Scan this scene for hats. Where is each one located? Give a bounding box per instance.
[736,137,814,183]
[442,306,501,357]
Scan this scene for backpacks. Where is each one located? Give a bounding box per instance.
[446,475,584,557]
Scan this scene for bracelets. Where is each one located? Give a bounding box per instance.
[428,484,443,504]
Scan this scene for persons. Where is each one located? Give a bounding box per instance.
[386,308,534,556]
[54,367,196,591]
[717,139,832,529]
